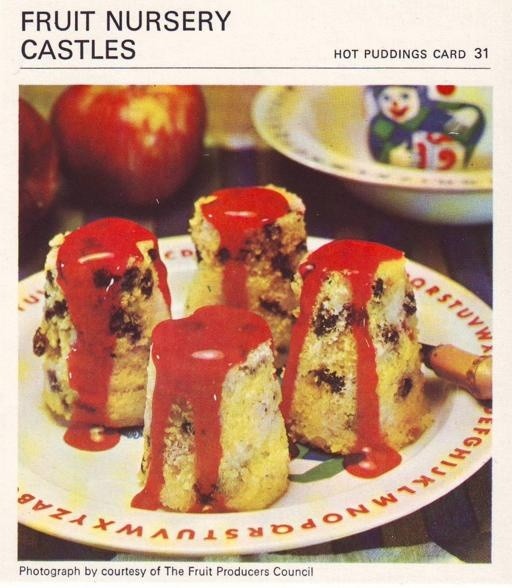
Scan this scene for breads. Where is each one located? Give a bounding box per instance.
[281,237,434,456]
[138,306,289,511]
[184,184,308,368]
[32,218,174,428]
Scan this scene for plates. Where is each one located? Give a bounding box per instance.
[17,235,495,555]
[249,88,486,226]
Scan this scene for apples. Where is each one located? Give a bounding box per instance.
[51,85,207,206]
[18,99,62,243]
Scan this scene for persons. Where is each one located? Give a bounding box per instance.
[366,85,487,170]
